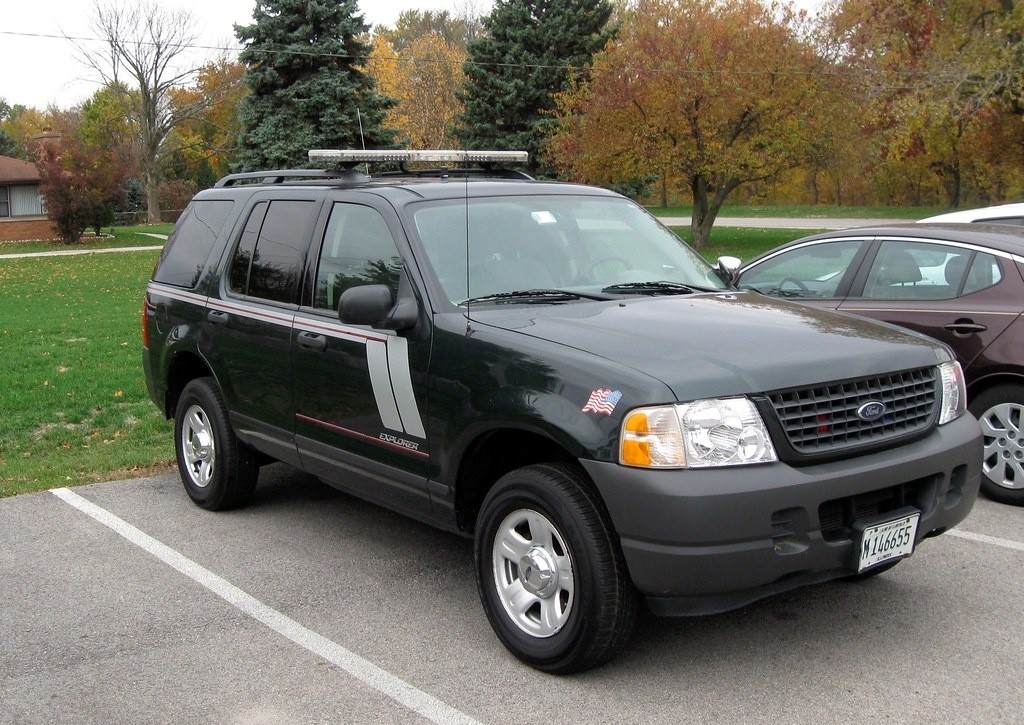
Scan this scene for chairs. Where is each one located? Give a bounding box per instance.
[480,220,550,290]
[882,251,924,299]
[943,256,977,297]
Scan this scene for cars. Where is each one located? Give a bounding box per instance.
[732,221,1024,512]
[813,200,1024,285]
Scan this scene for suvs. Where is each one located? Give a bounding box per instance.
[139,151,985,678]
